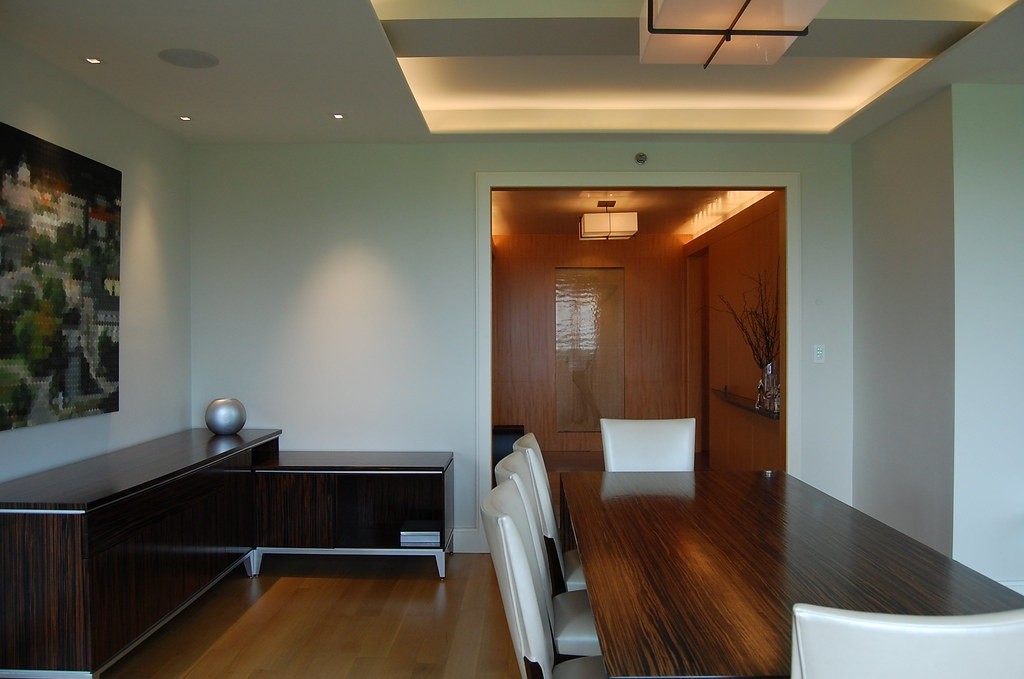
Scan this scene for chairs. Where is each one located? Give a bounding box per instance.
[480,480,610,679]
[599,416,696,470]
[512,434,586,590]
[791,601,1024,679]
[495,449,600,655]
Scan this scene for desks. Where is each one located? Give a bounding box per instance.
[559,466,1024,679]
[0,428,453,679]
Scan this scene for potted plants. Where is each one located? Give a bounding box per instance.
[718,265,781,412]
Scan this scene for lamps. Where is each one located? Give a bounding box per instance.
[578,201,639,240]
[638,0,825,71]
